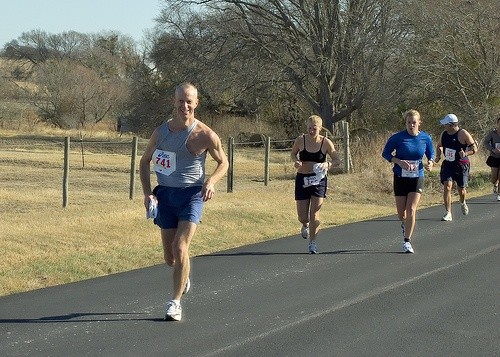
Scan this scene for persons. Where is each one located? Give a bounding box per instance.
[140,83,230,321]
[484,117,500,201]
[435,114,478,221]
[382,110,434,253]
[290,115,340,254]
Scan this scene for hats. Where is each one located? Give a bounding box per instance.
[440,114,458,125]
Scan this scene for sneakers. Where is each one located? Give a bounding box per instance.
[184,278,191,294]
[493,183,500,200]
[401,240,415,255]
[308,240,317,254]
[301,224,309,238]
[442,210,452,222]
[165,297,182,321]
[401,222,406,237]
[460,199,468,215]
